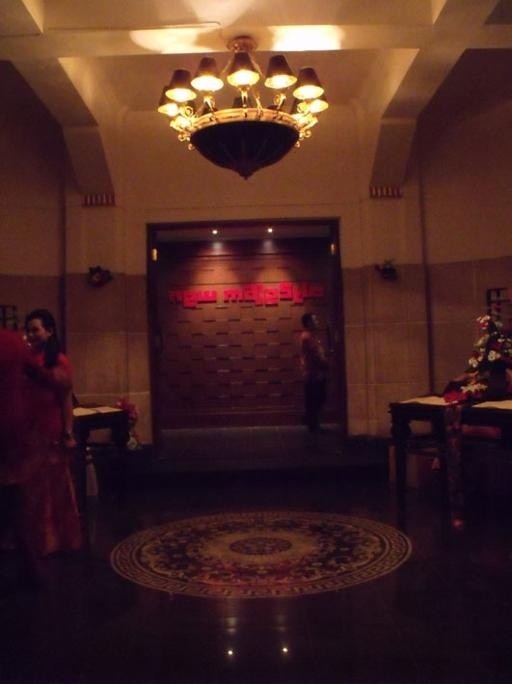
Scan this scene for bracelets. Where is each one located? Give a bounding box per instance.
[64,432,73,438]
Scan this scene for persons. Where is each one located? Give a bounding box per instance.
[1,325,72,607]
[23,307,84,555]
[299,310,333,433]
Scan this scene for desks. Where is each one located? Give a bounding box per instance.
[389,393,512,535]
[71,402,131,512]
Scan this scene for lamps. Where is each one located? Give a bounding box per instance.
[155,37,333,179]
